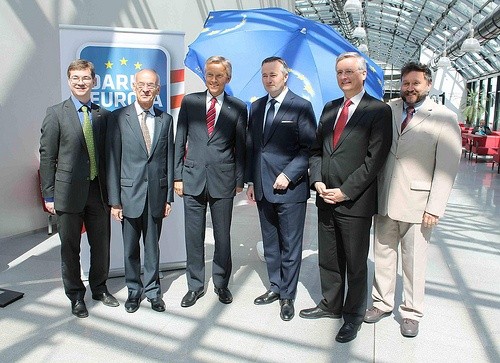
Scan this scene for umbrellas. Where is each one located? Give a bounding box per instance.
[183,7,385,121]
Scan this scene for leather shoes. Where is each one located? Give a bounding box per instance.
[364,306,392,323]
[299,305,342,319]
[71,299,89,318]
[335,321,362,343]
[214,286,233,304]
[181,290,204,307]
[401,318,419,337]
[254,290,279,305]
[124,297,140,313]
[92,289,120,307]
[147,297,166,312]
[280,296,294,321]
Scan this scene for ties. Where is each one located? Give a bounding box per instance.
[333,99,352,149]
[206,98,217,136]
[140,111,151,155]
[82,105,98,181]
[262,99,278,142]
[401,107,415,132]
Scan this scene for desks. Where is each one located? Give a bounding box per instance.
[462,133,486,160]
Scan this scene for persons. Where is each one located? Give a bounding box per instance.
[109,69,175,313]
[174,55,248,307]
[39,60,120,318]
[471,119,492,135]
[243,56,317,320]
[365,62,462,336]
[299,52,393,343]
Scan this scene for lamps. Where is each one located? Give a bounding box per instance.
[358,33,368,53]
[344,0,364,12]
[461,0,482,53]
[352,9,366,38]
[439,17,451,67]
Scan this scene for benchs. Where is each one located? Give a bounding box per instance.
[457,121,500,175]
